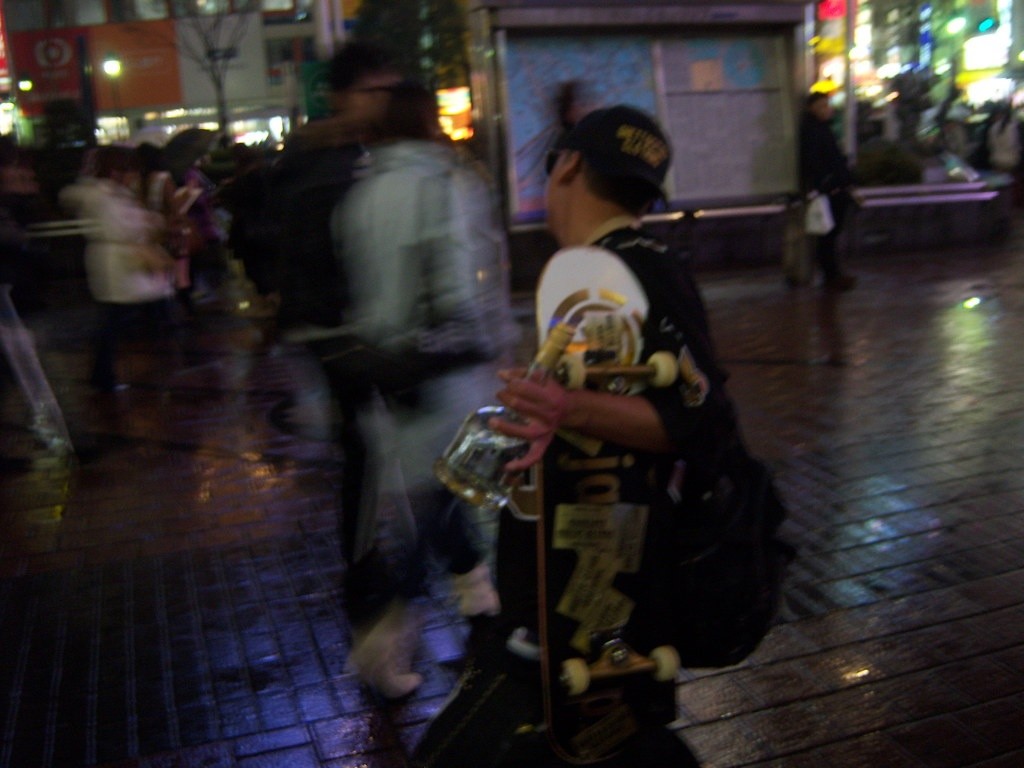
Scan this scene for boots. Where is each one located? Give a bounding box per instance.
[349,618,423,698]
[451,559,501,616]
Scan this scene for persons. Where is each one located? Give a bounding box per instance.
[329,74,524,703]
[0,84,1024,401]
[400,104,722,767]
[261,27,441,684]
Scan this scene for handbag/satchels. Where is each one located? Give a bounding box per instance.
[629,450,793,669]
[803,194,832,234]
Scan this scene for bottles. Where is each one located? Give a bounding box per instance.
[429,324,577,512]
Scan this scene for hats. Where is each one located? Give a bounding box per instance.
[553,105,673,219]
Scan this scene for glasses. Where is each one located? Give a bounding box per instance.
[546,151,560,176]
